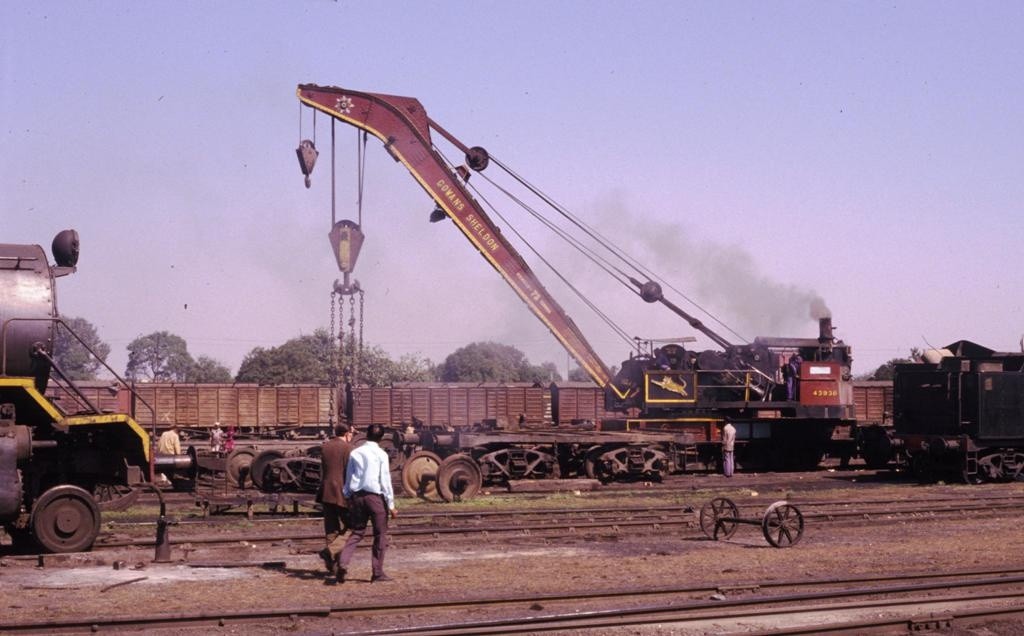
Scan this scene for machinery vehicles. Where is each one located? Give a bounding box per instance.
[294,82,855,472]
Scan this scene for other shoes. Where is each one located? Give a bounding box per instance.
[319,548,337,574]
[337,565,348,584]
[372,574,392,581]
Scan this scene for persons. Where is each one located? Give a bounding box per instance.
[315,423,398,583]
[722,415,736,477]
[785,355,802,401]
[156,422,235,457]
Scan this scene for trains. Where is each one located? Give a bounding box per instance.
[42,341,1024,487]
[0,230,174,564]
